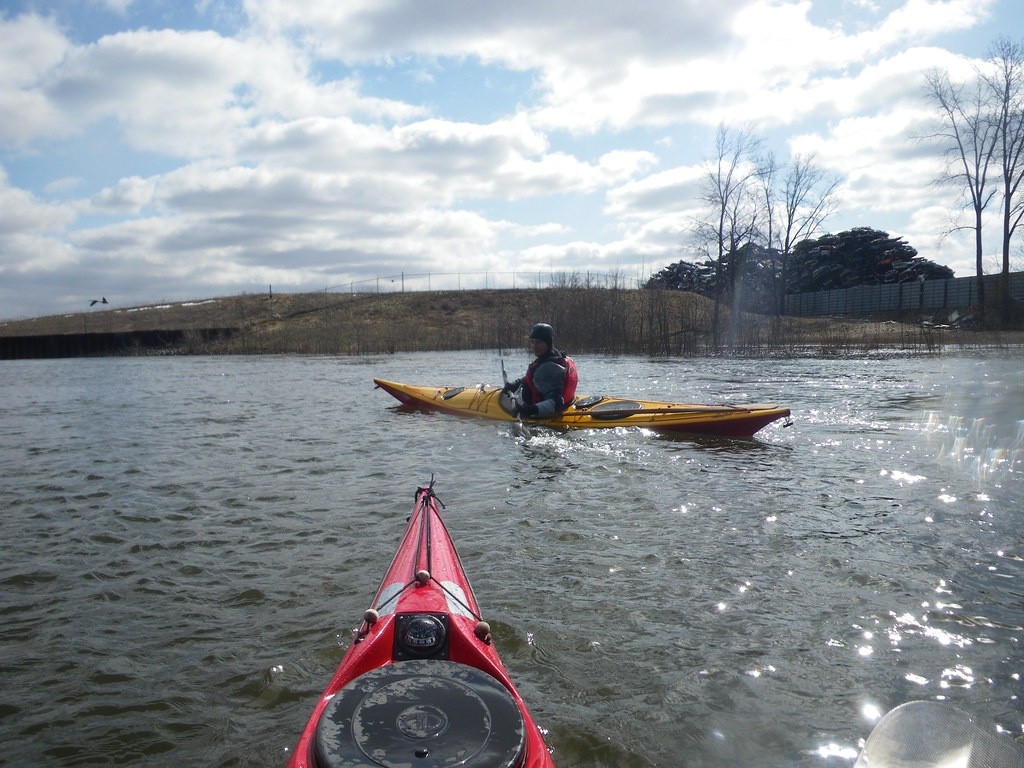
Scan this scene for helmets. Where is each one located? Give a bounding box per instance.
[529,322,554,347]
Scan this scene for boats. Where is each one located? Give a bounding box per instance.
[373,377,793,438]
[285,481,559,768]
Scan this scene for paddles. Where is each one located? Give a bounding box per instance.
[563,402,778,416]
[501,358,531,437]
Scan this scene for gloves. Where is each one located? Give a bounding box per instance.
[518,403,537,416]
[505,379,520,394]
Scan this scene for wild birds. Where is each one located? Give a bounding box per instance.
[95,297,109,304]
[88,300,97,306]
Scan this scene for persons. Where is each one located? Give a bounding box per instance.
[502,322,569,418]
